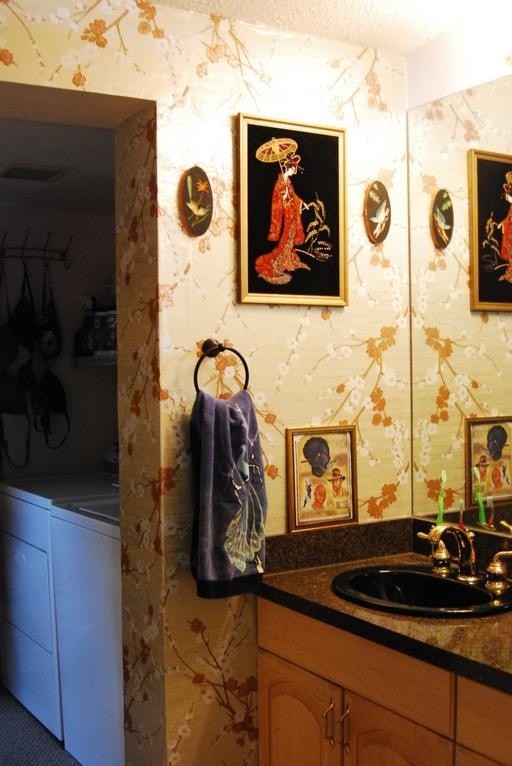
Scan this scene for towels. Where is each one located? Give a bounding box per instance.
[189,391,268,599]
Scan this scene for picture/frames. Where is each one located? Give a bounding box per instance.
[468,149,512,313]
[464,416,512,512]
[286,425,359,533]
[238,114,348,307]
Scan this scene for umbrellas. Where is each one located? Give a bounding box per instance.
[254,134,301,176]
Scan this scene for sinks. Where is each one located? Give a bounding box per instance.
[331,563,512,619]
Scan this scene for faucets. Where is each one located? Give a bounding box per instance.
[425,523,481,577]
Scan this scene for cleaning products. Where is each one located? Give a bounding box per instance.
[80,297,99,354]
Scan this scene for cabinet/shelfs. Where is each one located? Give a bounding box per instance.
[455,675,512,766]
[257,596,455,766]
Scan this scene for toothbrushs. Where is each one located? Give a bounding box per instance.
[458,499,465,528]
[436,470,446,524]
[471,466,486,524]
[486,496,493,527]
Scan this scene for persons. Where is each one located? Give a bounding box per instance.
[491,467,508,489]
[310,486,327,511]
[256,150,314,288]
[485,425,511,486]
[299,436,335,512]
[496,168,512,285]
[476,455,490,481]
[326,468,351,512]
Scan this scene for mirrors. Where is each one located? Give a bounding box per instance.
[406,76,512,536]
